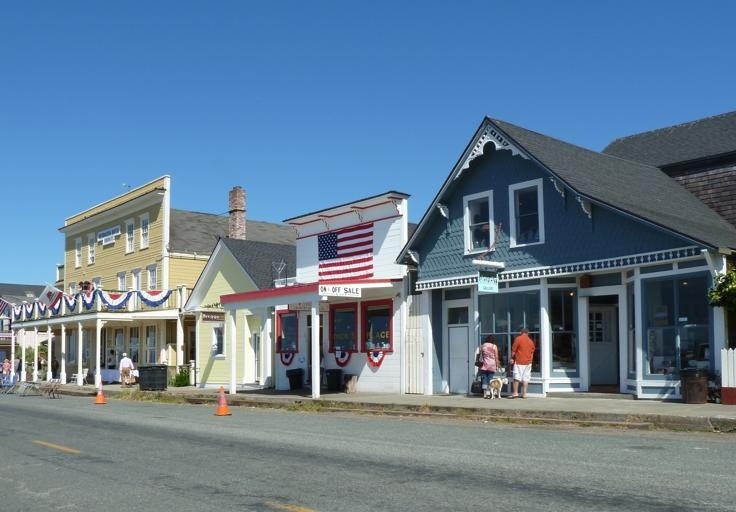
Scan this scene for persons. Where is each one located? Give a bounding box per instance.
[106,349,116,369]
[478,335,499,399]
[52,357,59,379]
[0,356,22,382]
[119,353,135,388]
[507,328,535,399]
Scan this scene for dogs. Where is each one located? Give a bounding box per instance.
[489,378,509,399]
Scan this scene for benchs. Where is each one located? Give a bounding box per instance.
[66,367,89,384]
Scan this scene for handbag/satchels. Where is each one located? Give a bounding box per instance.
[471,372,483,394]
[475,346,484,367]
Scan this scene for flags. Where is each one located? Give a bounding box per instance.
[0,299,8,317]
[317,223,374,285]
[37,285,64,311]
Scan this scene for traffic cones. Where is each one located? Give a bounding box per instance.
[95,379,107,404]
[216,386,232,415]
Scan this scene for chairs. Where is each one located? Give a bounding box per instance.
[38,378,63,399]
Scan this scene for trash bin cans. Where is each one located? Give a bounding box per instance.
[138,366,152,391]
[150,364,167,391]
[679,367,711,405]
